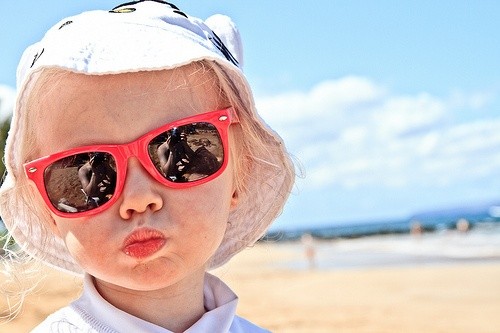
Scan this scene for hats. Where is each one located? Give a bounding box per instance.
[0,1,296,276]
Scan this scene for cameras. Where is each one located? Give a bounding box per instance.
[170,128,182,143]
[90,153,103,164]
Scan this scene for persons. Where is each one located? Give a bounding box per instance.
[79,153,115,197]
[0,0,300,332]
[156,127,197,178]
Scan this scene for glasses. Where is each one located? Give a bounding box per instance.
[23,107,239,217]
